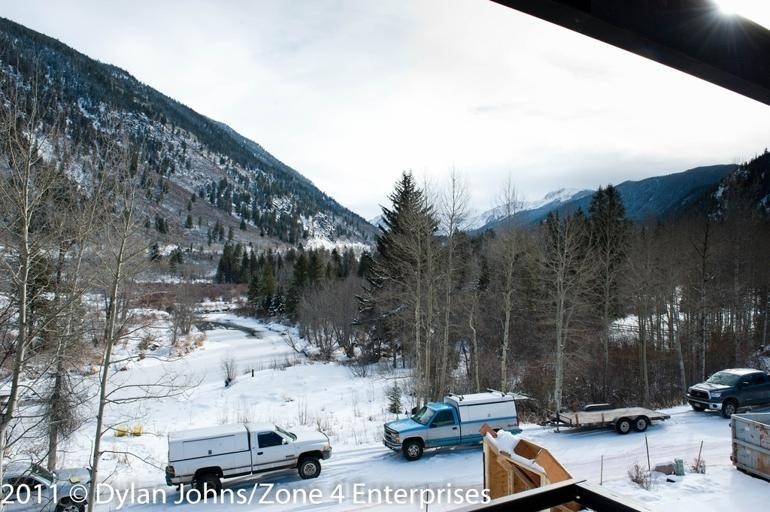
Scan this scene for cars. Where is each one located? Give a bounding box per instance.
[0,458,97,512]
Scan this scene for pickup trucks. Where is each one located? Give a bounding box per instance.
[383,390,522,461]
[164,421,334,498]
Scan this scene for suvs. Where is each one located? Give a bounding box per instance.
[683,367,770,420]
